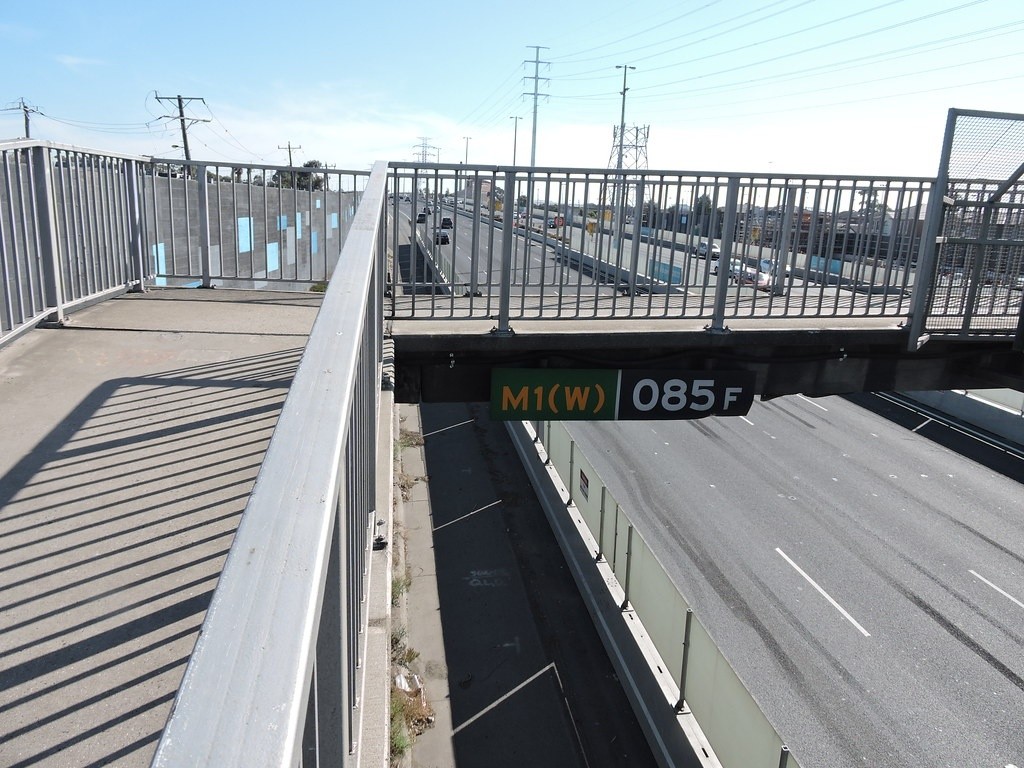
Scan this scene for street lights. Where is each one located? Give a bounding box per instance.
[463,137,472,212]
[613,66,636,249]
[510,116,523,166]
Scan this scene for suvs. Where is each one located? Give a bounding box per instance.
[436,232,449,244]
[441,218,453,228]
[405,197,411,202]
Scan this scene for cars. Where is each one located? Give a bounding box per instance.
[548,218,556,228]
[694,242,721,259]
[514,211,531,219]
[900,259,1024,291]
[427,197,463,205]
[417,206,440,223]
[714,257,791,291]
